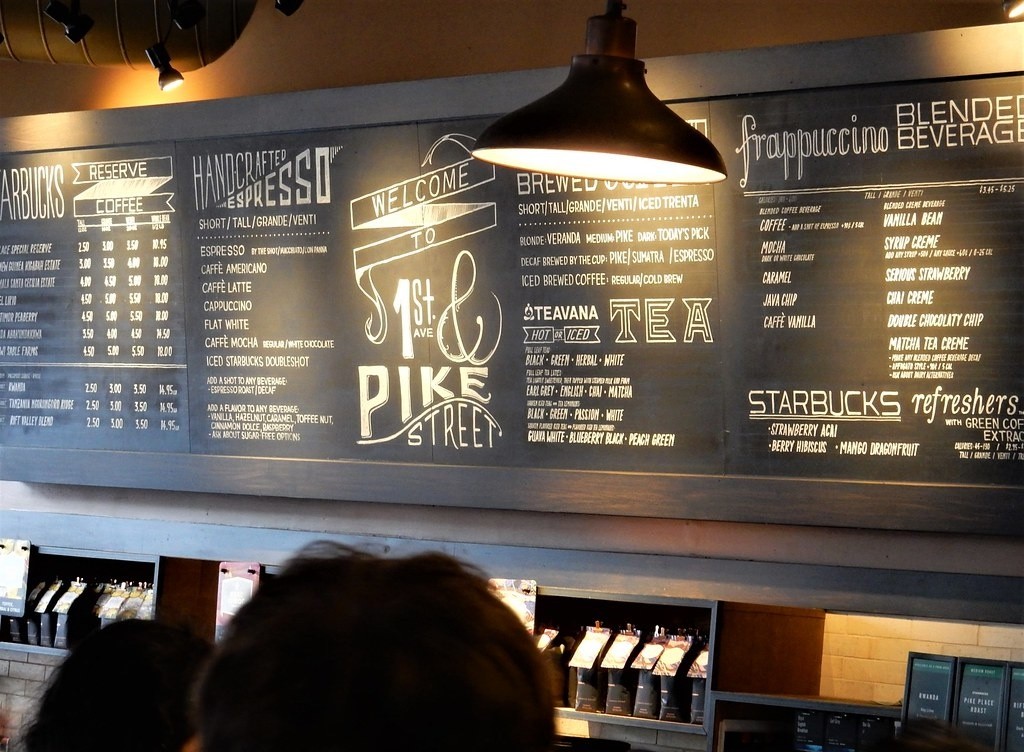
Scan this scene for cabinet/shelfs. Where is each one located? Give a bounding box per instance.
[0,540,1024,751]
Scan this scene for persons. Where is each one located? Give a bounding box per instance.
[22,541,558,752]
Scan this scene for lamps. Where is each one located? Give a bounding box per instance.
[146,44,186,91]
[473,0,727,183]
[43,1,95,45]
[275,0,304,16]
